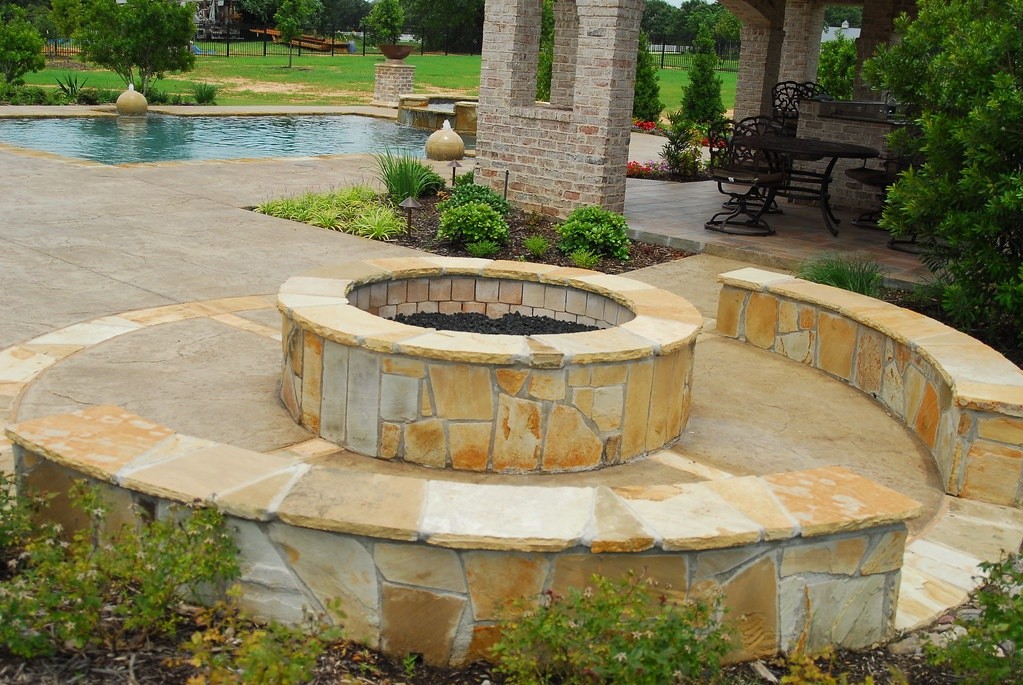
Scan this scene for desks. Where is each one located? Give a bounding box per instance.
[734,135,880,237]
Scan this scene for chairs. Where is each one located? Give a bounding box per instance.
[706,81,834,238]
[845,131,963,260]
